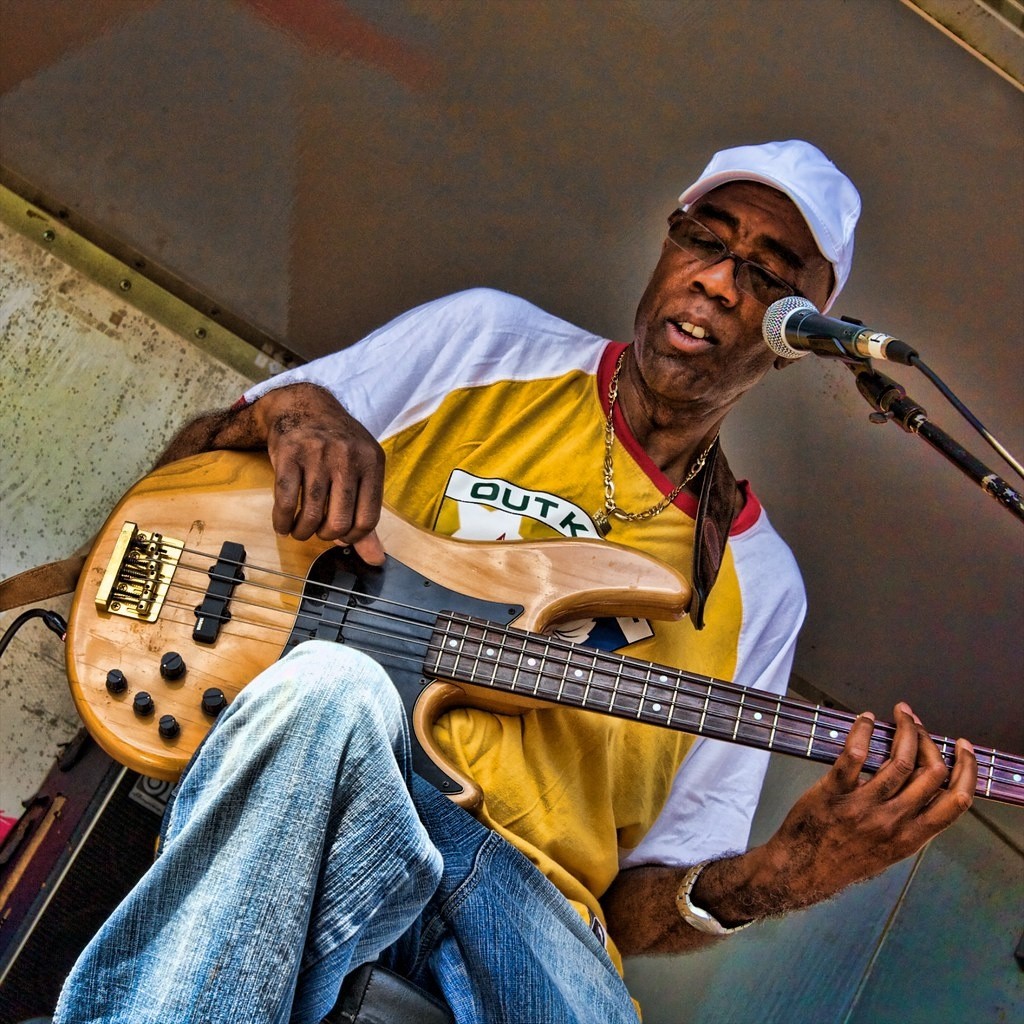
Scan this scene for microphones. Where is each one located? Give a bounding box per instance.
[762,296,919,367]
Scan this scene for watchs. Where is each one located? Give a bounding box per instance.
[673,847,769,937]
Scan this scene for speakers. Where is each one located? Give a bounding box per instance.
[0,742,176,1024]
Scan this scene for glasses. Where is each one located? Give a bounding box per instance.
[667,208,805,312]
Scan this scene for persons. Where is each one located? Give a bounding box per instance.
[52,136,980,1024]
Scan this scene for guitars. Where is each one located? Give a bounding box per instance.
[62,440,1023,815]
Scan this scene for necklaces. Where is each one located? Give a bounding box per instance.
[591,351,720,537]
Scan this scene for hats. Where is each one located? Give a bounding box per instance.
[680,139,862,316]
